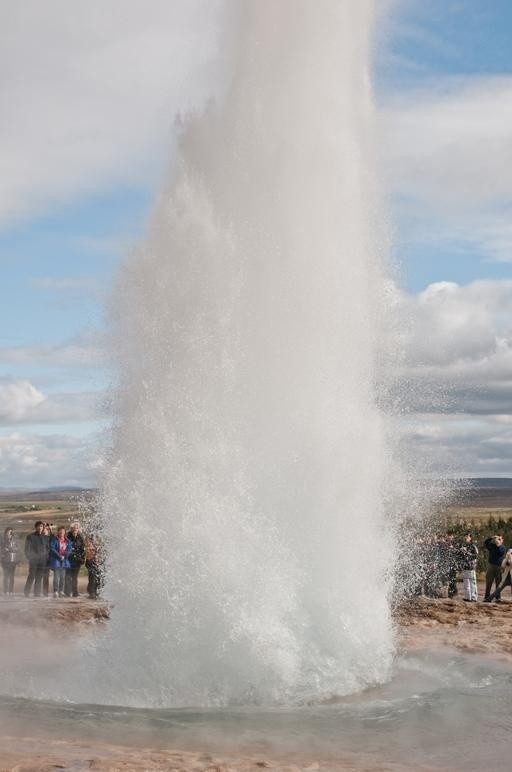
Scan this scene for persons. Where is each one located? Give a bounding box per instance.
[83,523,105,598]
[42,521,56,596]
[397,528,479,603]
[23,520,51,598]
[482,532,504,602]
[1,526,21,597]
[482,549,511,604]
[64,521,86,598]
[49,526,72,598]
